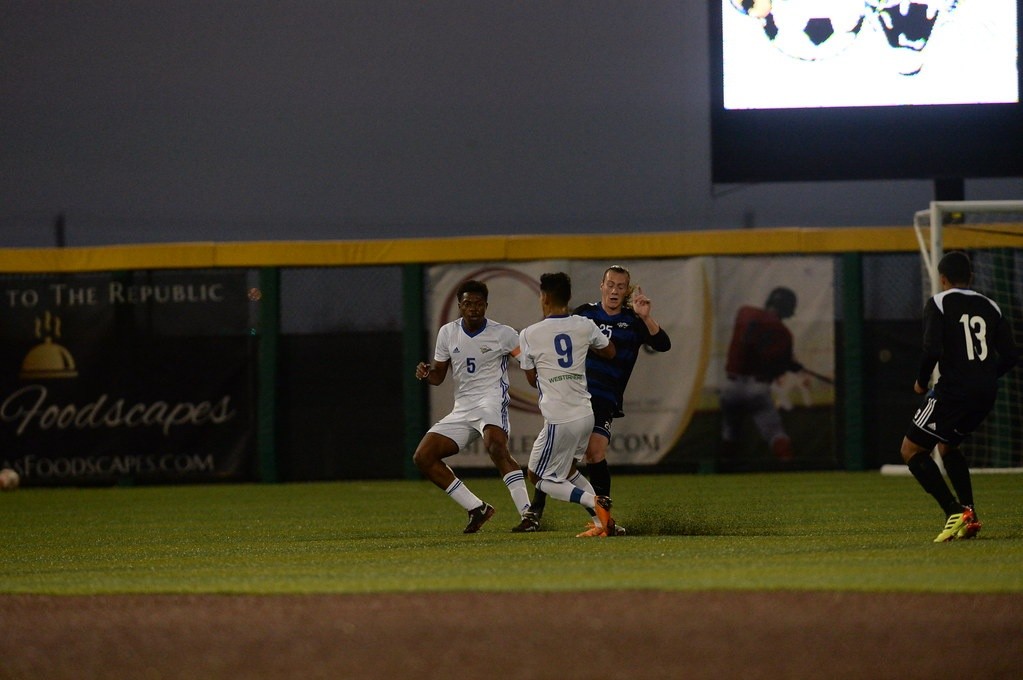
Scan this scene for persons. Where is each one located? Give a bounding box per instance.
[519,272,628,536]
[718,288,800,473]
[412,280,532,534]
[901,252,1019,543]
[512,267,670,536]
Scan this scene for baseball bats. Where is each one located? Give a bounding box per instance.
[801,368,832,384]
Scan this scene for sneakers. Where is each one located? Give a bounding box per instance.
[934,506,973,543]
[511,512,541,533]
[576,526,604,538]
[609,524,625,536]
[594,496,613,537]
[463,502,495,533]
[956,521,981,539]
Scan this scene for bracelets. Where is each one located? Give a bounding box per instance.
[423,370,429,377]
